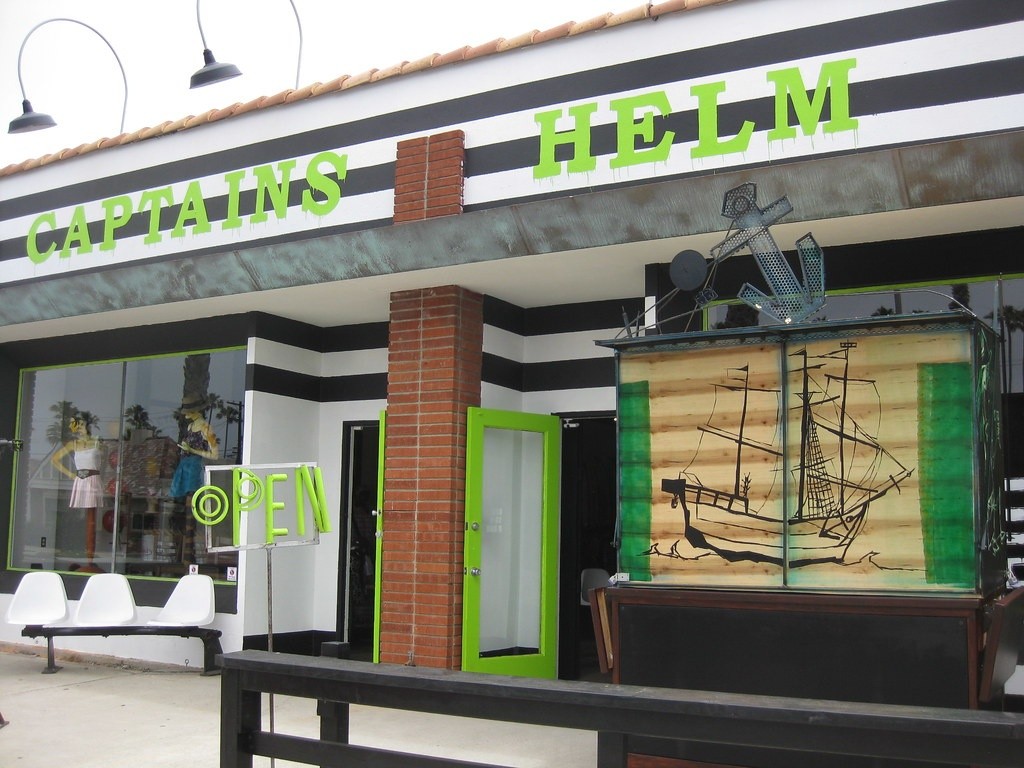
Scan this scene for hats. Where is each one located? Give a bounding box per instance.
[180,393,210,413]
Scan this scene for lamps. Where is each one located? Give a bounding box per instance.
[8,18,127,136]
[189,0,302,91]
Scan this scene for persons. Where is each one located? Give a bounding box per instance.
[167,389,219,497]
[51,412,105,507]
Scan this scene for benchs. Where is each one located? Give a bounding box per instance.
[4,572,226,677]
